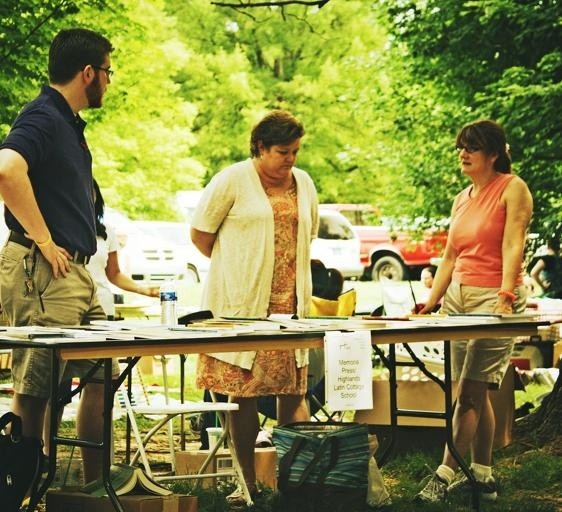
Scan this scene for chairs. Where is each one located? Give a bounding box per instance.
[380,276,415,315]
[114,356,254,507]
[261,288,356,427]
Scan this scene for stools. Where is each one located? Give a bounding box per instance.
[175,449,232,490]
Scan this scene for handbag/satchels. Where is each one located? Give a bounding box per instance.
[272,419,371,503]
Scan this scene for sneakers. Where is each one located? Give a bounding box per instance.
[411,471,449,505]
[448,467,498,501]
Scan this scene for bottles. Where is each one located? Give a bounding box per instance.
[160,276,178,331]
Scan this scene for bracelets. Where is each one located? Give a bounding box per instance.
[35,233,52,246]
[497,291,517,302]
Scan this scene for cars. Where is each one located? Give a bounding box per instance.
[309,204,449,283]
[103,206,211,306]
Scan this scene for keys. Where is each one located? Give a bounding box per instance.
[23,272,35,296]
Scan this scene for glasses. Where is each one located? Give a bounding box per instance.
[81,66,114,76]
[456,144,483,153]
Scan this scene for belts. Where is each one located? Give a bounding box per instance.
[10,231,92,265]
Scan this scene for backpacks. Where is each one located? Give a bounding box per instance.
[0,411,44,512]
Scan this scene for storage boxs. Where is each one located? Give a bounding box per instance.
[254,446,278,492]
[178,494,198,512]
[514,338,562,368]
[46,490,180,512]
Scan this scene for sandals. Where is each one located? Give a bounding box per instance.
[226,484,257,503]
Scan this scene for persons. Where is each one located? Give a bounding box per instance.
[413,120,534,502]
[191,113,319,509]
[1,30,123,510]
[38,179,165,473]
[529,233,561,300]
[198,258,345,451]
[413,266,443,314]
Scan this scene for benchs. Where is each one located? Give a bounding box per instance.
[354,355,515,453]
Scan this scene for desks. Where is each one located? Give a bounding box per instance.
[292,315,560,511]
[0,319,341,512]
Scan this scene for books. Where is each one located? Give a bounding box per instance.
[81,462,173,498]
[1,308,547,346]
